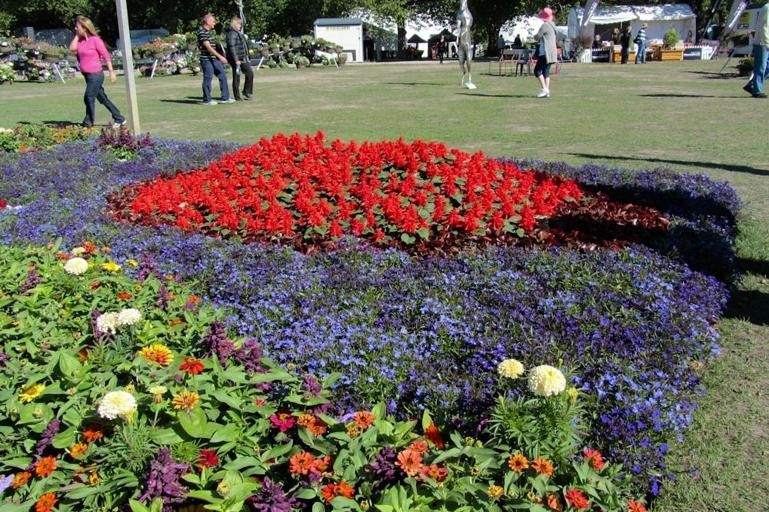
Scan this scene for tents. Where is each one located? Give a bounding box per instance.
[497,0,697,67]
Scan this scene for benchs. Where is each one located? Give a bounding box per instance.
[488,48,562,77]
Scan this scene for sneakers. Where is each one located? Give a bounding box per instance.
[536,87,551,97]
[203,92,253,106]
[744,84,767,97]
[113,120,126,129]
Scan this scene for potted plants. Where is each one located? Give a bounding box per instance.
[132,31,201,76]
[660,27,685,61]
[260,33,347,69]
[0,35,77,86]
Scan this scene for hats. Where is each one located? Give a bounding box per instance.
[538,7,553,20]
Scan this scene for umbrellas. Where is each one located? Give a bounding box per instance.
[407,33,427,51]
[428,28,457,43]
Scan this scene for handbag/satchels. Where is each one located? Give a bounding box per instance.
[532,42,540,59]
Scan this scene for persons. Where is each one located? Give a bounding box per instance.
[198,15,237,107]
[534,7,557,101]
[453,0,475,91]
[433,37,449,64]
[225,15,254,103]
[742,3,769,99]
[67,15,126,131]
[451,44,458,59]
